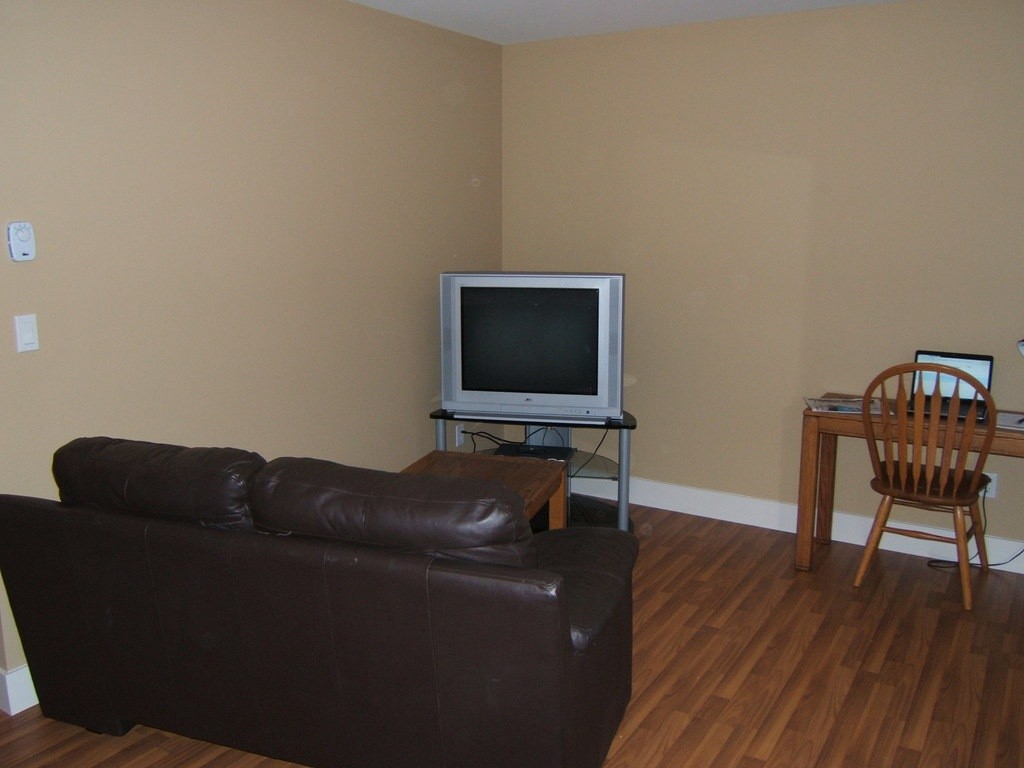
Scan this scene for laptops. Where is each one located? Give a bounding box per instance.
[905,350,993,422]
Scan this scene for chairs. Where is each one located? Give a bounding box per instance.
[850,362,998,611]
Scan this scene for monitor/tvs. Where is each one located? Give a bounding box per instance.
[440,271,624,426]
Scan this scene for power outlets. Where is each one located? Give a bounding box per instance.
[978,472,998,498]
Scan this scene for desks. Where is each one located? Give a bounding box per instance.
[398,408,637,533]
[794,393,1024,573]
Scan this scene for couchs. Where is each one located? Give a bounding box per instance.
[0,430,640,768]
